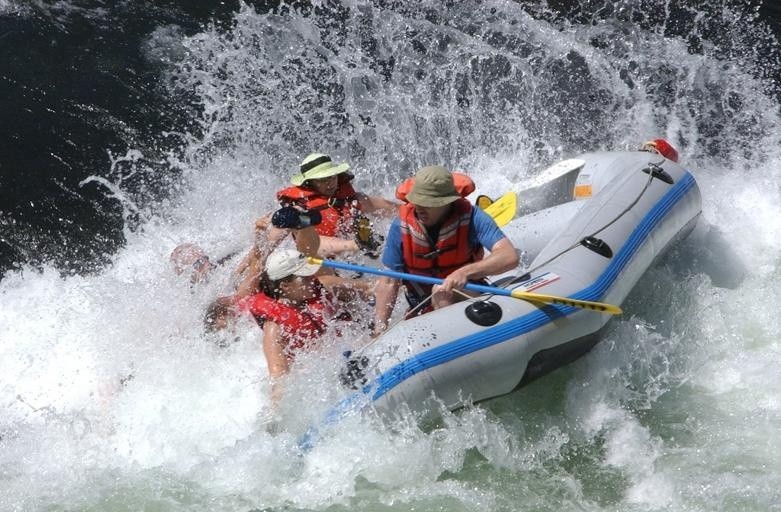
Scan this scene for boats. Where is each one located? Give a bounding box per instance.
[299,150,703,450]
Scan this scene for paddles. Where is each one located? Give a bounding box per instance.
[477,190,517,227]
[308,257,622,315]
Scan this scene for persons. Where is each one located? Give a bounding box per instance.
[171,153,521,405]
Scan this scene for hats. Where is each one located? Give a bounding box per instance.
[271,206,323,231]
[405,164,463,208]
[290,152,350,187]
[266,249,324,282]
